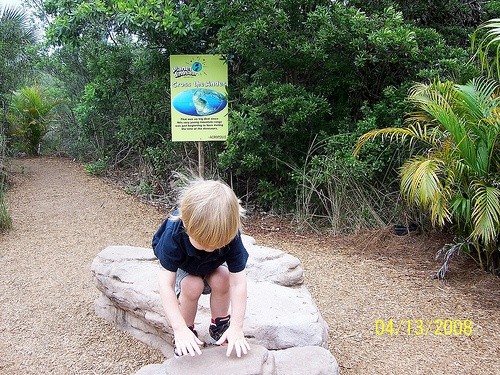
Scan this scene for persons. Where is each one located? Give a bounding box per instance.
[152,180,251,358]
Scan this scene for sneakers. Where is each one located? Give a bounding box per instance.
[172,324,199,359]
[208,314,231,346]
